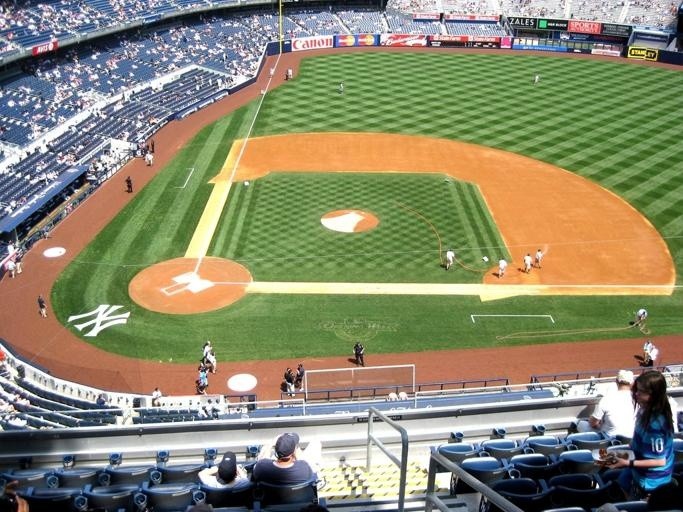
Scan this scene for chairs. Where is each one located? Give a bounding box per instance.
[437,411,683,512]
[0,340,327,512]
[267,1,682,65]
[0,1,268,261]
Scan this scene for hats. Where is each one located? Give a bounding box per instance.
[215,450,238,484]
[616,369,635,385]
[273,429,301,460]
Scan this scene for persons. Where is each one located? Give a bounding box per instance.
[0,0,336,278]
[197,341,217,394]
[647,344,658,367]
[152,387,162,406]
[533,73,539,87]
[96,394,105,404]
[336,0,681,52]
[339,82,344,96]
[198,451,248,488]
[533,249,543,269]
[251,433,314,486]
[595,370,674,502]
[2,479,29,511]
[284,364,305,397]
[638,308,648,327]
[643,339,652,364]
[2,350,31,431]
[351,340,365,368]
[444,248,455,269]
[498,258,508,278]
[37,294,48,318]
[589,369,641,446]
[203,341,212,364]
[577,399,595,425]
[523,253,532,273]
[197,398,221,420]
[666,395,679,433]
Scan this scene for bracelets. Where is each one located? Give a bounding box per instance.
[630,460,633,467]
